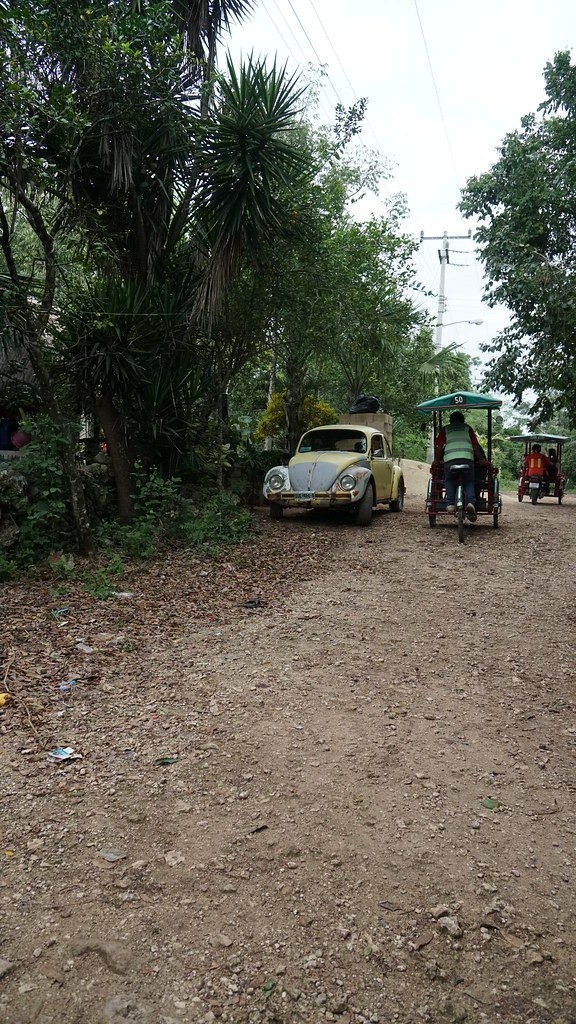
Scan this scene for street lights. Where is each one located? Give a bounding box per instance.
[442,319,484,328]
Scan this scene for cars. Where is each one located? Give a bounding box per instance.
[263,423,406,528]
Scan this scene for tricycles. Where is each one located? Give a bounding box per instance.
[426,458,502,543]
[517,465,568,506]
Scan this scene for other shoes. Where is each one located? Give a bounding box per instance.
[445,504,455,513]
[467,503,477,522]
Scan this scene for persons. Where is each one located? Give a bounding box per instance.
[524,444,558,493]
[435,411,487,522]
